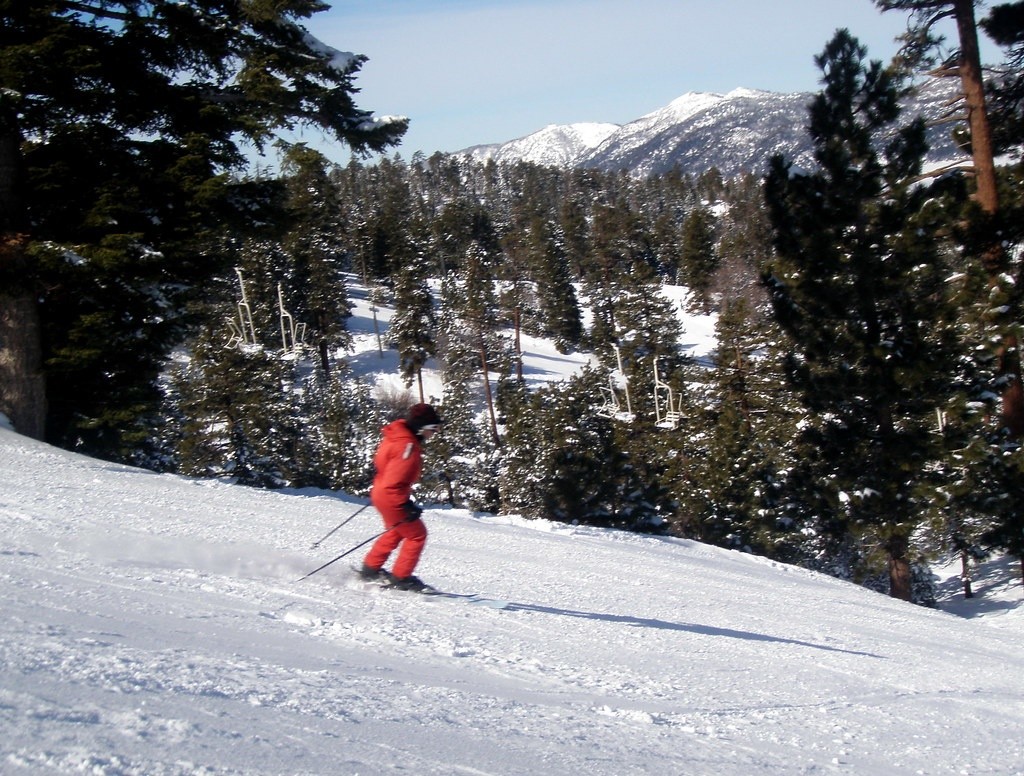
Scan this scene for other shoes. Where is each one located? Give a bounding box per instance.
[389,575,422,590]
[361,566,389,578]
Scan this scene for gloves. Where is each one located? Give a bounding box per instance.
[401,500,423,522]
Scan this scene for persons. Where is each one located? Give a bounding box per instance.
[361,403,440,586]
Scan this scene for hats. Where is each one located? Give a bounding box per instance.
[406,403,447,430]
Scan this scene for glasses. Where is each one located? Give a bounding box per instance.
[419,423,444,432]
